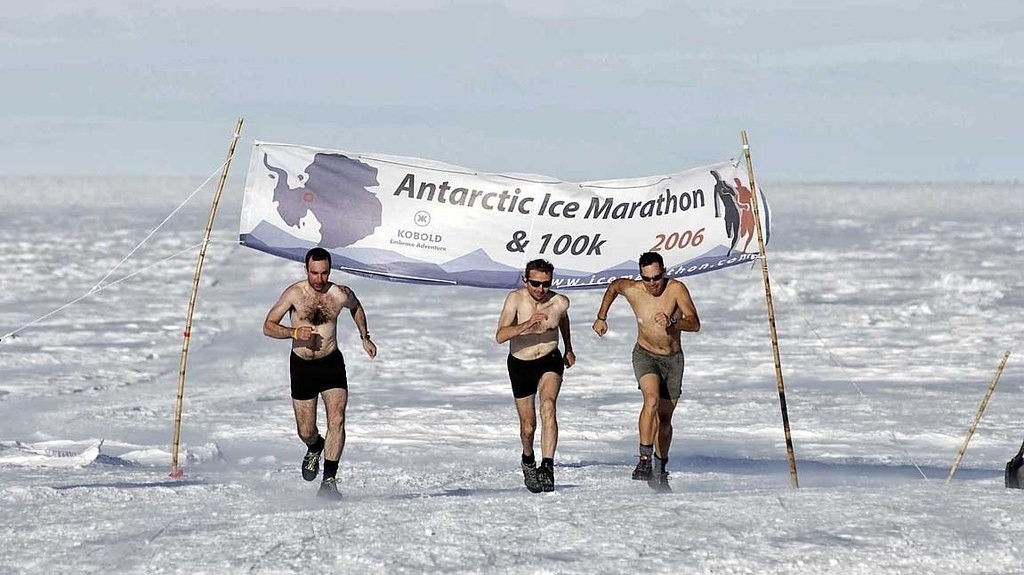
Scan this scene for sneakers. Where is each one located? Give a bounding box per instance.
[632,455,654,480]
[648,471,673,493]
[317,477,343,499]
[302,438,325,480]
[537,464,555,492]
[521,460,541,493]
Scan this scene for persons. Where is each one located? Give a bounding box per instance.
[496,259,576,495]
[264,247,377,501]
[591,252,700,494]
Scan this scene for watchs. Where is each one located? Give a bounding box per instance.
[667,316,677,328]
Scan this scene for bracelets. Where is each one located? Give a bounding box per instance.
[294,327,302,340]
[597,314,607,321]
[360,334,371,340]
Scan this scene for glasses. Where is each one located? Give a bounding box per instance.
[641,272,664,282]
[527,279,552,287]
